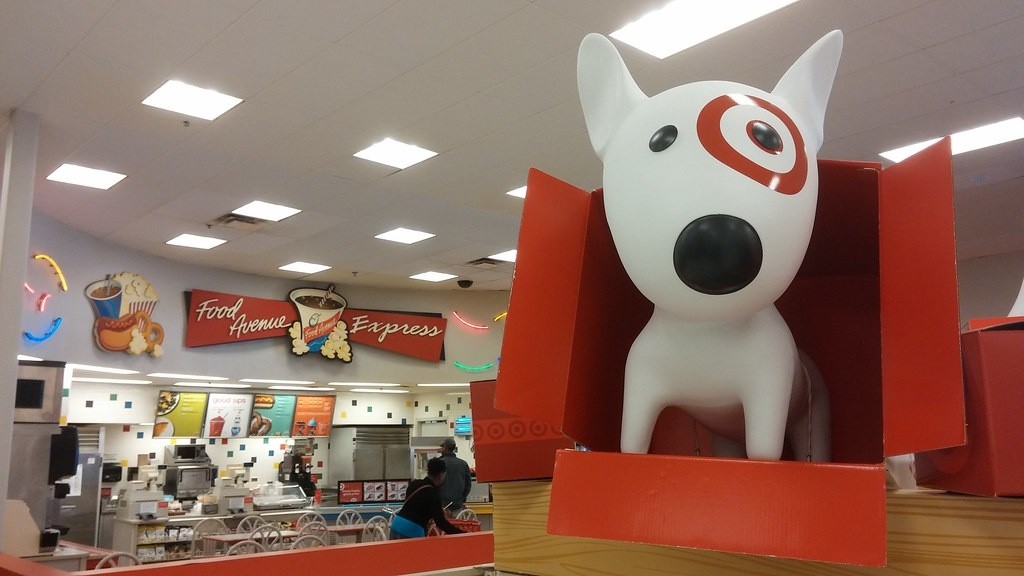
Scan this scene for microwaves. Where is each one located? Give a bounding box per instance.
[173,444,206,463]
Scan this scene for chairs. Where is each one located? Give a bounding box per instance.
[427,523,447,536]
[94,508,402,570]
[456,508,477,520]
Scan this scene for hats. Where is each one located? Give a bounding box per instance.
[440,438,456,450]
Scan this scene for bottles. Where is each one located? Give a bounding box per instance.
[455,415,471,434]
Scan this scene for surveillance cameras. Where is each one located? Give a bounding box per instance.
[457,280,474,288]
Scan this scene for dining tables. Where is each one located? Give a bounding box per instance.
[311,523,392,546]
[202,530,310,558]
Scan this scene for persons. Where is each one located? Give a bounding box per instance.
[290,455,317,496]
[434,440,471,515]
[390,457,468,540]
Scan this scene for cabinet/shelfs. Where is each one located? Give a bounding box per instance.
[112,509,312,565]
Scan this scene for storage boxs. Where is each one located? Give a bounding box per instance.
[497,136,965,568]
[470,380,576,483]
[914,320,1024,496]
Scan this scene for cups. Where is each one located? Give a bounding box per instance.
[290,289,346,352]
[86,279,122,319]
